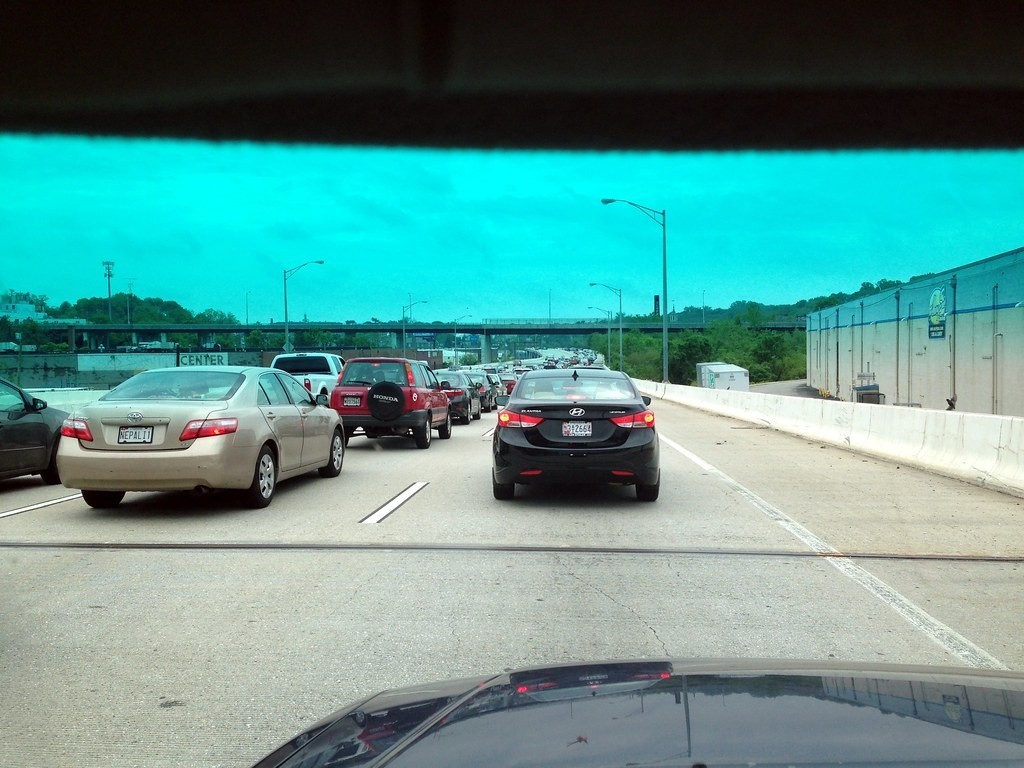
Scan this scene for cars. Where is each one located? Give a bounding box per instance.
[497,348,598,381]
[424,369,482,425]
[0,377,72,486]
[486,374,508,397]
[55,365,346,509]
[492,368,661,502]
[500,373,518,396]
[456,369,499,413]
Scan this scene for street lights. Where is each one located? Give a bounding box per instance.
[408,293,412,323]
[246,291,252,327]
[548,287,552,324]
[589,306,611,369]
[590,282,623,373]
[403,299,427,359]
[702,290,706,324]
[602,198,675,385]
[285,260,325,352]
[455,315,473,364]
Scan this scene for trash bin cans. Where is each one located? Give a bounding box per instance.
[851,383,880,404]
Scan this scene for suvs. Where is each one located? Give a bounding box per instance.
[265,352,347,407]
[330,356,453,449]
